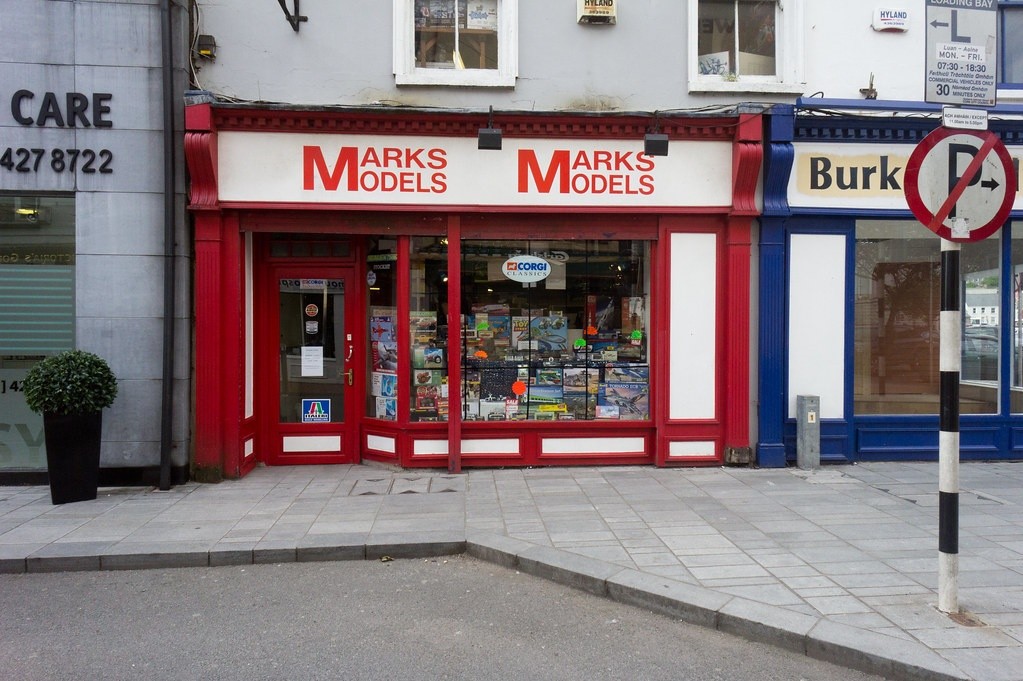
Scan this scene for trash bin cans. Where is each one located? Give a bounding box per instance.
[796,394,820,471]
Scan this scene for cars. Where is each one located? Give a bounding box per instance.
[428,349,443,363]
[854,297,998,386]
[416,318,435,329]
[414,335,434,344]
[964,324,999,352]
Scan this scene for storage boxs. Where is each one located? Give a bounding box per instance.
[369,296,650,422]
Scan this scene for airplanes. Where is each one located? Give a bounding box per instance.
[602,389,645,415]
[372,321,389,340]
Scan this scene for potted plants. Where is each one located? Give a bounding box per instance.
[25,350,117,505]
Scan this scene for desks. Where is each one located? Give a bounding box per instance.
[415,26,493,69]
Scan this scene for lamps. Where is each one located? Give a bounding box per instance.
[478,105,502,150]
[644,110,668,156]
